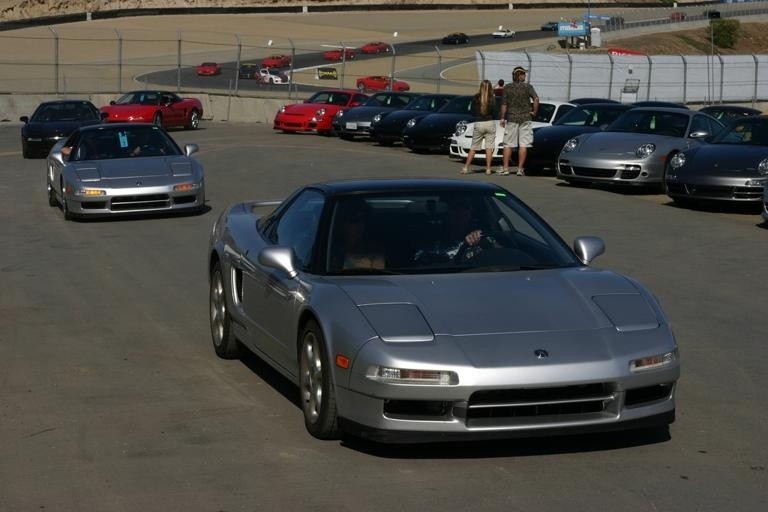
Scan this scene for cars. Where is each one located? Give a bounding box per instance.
[20,100,108,160]
[258,69,290,84]
[666,114,768,202]
[263,55,291,67]
[704,10,720,19]
[333,90,429,139]
[207,178,681,438]
[403,95,474,153]
[356,74,409,91]
[239,64,260,78]
[448,99,594,164]
[698,105,765,128]
[606,17,625,26]
[99,90,203,130]
[197,62,221,75]
[275,91,382,137]
[524,97,689,176]
[47,125,205,215]
[369,94,458,146]
[671,12,688,20]
[557,107,744,191]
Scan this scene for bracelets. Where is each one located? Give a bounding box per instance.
[532,110,537,114]
[501,116,505,119]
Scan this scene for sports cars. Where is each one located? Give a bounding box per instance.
[540,21,558,31]
[361,41,390,54]
[442,33,470,45]
[323,49,356,61]
[491,28,516,39]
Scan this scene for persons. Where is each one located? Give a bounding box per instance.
[431,199,517,265]
[495,65,542,175]
[61,138,103,160]
[310,196,393,272]
[493,79,506,96]
[462,80,500,176]
[129,129,166,156]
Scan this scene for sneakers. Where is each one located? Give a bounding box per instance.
[496,168,509,175]
[485,169,492,175]
[459,167,470,174]
[517,168,525,176]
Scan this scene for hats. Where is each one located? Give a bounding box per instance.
[512,66,529,74]
